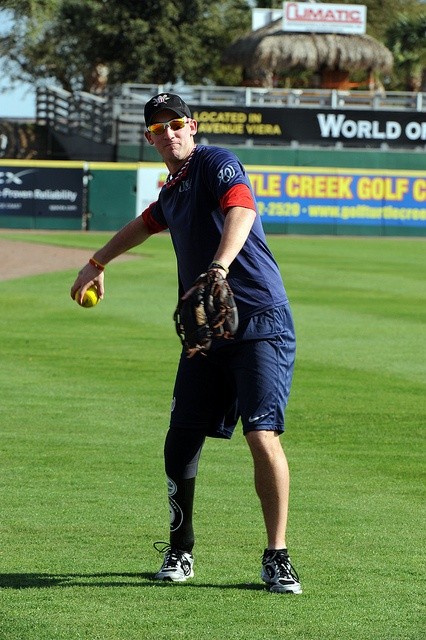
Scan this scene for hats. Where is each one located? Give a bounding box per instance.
[143,93,193,127]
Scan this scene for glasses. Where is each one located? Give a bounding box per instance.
[145,115,188,137]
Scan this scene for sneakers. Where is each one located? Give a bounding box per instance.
[260,547,303,595]
[152,541,195,581]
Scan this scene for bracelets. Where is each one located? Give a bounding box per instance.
[89,258,105,270]
[207,260,229,274]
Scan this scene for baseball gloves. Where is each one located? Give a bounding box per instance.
[174,270,239,369]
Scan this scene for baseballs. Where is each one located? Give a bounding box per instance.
[74,285,100,307]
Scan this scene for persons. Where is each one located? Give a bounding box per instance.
[70,93,303,595]
